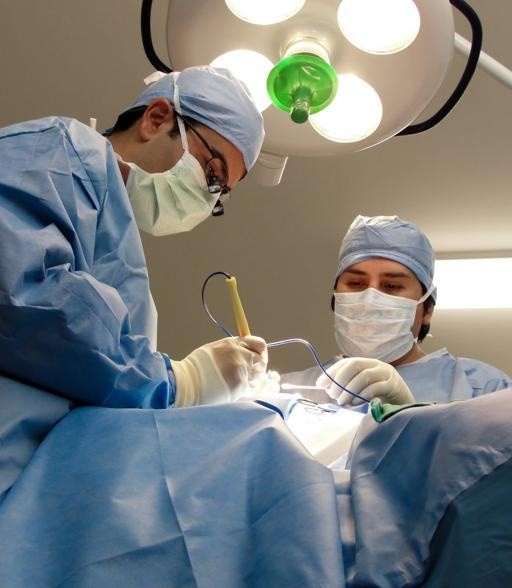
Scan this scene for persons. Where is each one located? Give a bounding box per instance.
[0,67,267,491]
[280,216,511,405]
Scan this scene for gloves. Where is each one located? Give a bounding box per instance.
[316,357,414,405]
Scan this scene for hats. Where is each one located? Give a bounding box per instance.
[328,216,438,305]
[120,66,264,181]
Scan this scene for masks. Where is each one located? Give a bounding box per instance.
[110,97,218,236]
[334,282,436,362]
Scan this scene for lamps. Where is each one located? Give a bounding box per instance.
[140,1,483,188]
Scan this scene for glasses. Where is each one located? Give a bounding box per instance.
[175,111,228,216]
